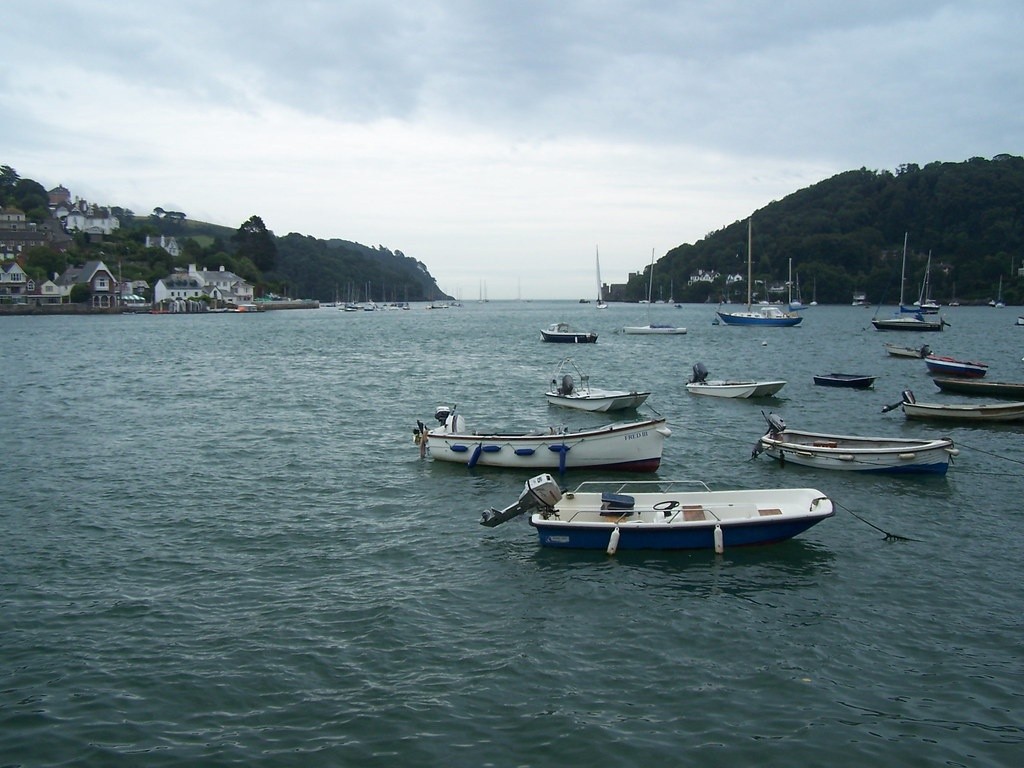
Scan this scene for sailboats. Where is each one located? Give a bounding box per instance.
[715,219,817,327]
[478,281,489,304]
[596,245,608,309]
[451,287,461,306]
[639,248,674,304]
[325,280,448,311]
[995,274,1006,308]
[871,231,960,330]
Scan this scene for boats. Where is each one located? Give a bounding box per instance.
[540,321,598,343]
[883,378,1024,421]
[545,357,651,413]
[851,291,866,305]
[989,300,995,305]
[413,402,667,472]
[813,372,877,389]
[479,474,835,551]
[757,410,953,474]
[623,323,687,335]
[883,340,988,378]
[685,362,787,399]
